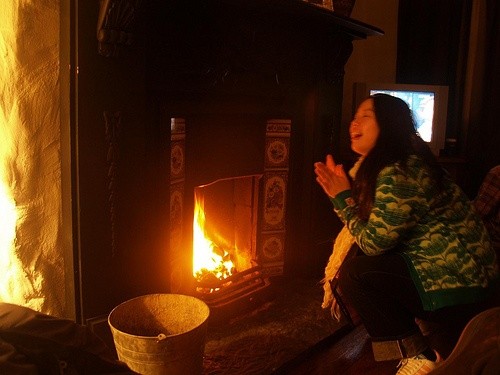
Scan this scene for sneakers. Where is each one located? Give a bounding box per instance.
[395,350,444,375]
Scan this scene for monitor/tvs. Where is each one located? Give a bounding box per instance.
[352,80,449,159]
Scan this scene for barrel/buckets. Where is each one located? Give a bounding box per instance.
[106,293,210,374]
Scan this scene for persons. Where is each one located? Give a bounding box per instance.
[314,93,500,375]
